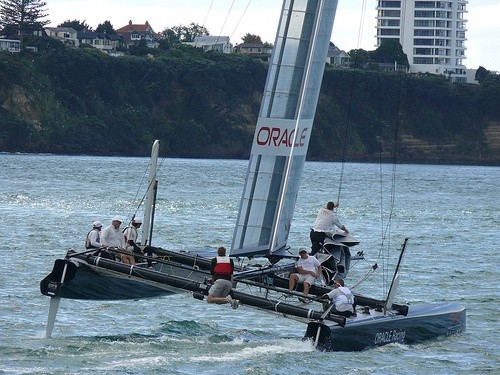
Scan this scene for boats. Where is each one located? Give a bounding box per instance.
[39,0,468,354]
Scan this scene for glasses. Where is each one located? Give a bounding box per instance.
[300,251,306,254]
[334,282,342,287]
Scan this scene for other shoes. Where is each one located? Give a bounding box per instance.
[226,295,233,304]
[230,299,240,309]
[284,293,293,298]
[298,297,308,304]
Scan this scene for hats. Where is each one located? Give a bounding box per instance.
[93,221,104,227]
[299,247,307,253]
[112,217,122,223]
[133,217,142,223]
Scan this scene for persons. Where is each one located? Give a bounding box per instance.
[100,216,136,265]
[122,217,145,256]
[85,221,112,259]
[308,202,349,256]
[314,279,356,318]
[206,247,240,309]
[289,247,321,296]
